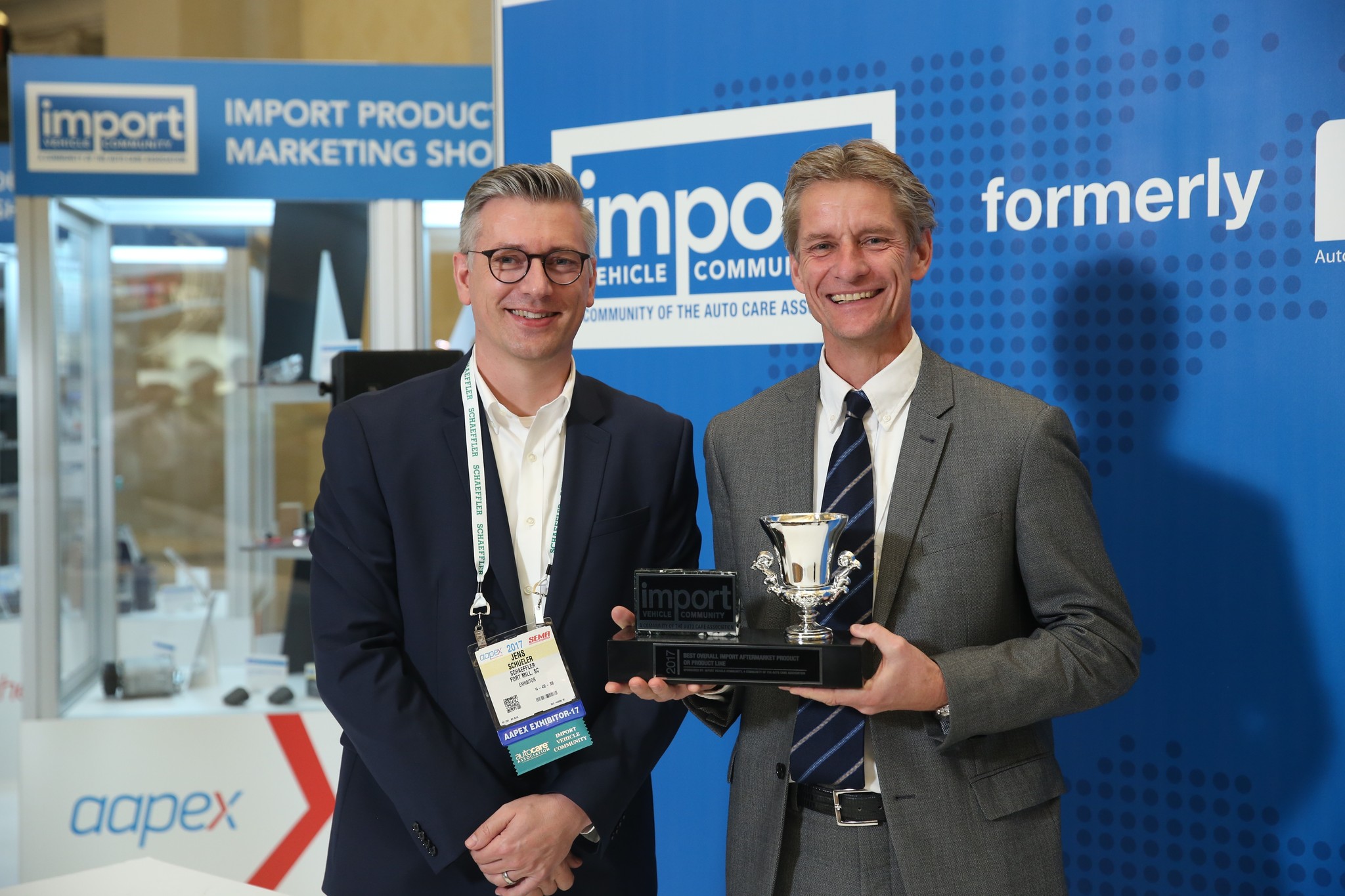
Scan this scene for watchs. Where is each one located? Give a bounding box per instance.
[934,704,951,722]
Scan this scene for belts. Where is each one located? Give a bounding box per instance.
[787,782,888,826]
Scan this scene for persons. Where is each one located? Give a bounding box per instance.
[603,144,1144,896]
[309,165,700,896]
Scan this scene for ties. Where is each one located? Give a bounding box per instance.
[789,389,875,789]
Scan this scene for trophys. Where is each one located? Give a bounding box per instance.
[606,512,878,689]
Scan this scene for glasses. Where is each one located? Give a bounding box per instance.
[466,247,590,285]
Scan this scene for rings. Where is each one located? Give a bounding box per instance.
[501,871,517,886]
